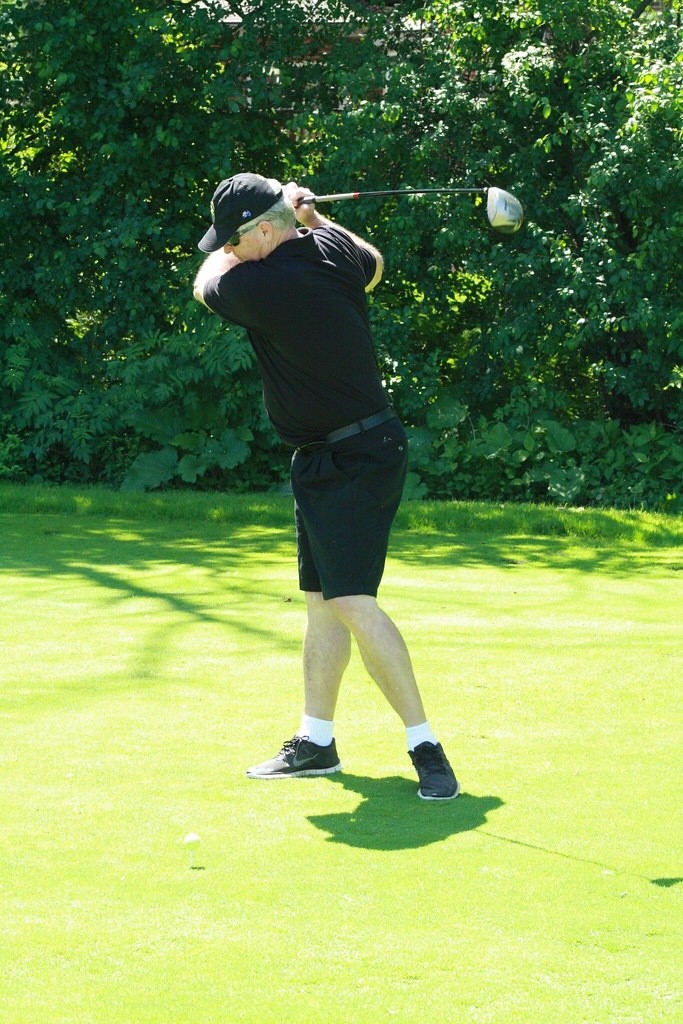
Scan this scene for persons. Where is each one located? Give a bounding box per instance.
[191,171,460,801]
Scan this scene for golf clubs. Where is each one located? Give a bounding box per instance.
[291,186,522,237]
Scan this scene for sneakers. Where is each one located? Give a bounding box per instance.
[245,736,341,779]
[407,741,460,801]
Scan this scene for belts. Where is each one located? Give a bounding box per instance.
[318,406,398,444]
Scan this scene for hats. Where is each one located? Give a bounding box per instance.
[198,173,283,253]
[227,220,268,246]
